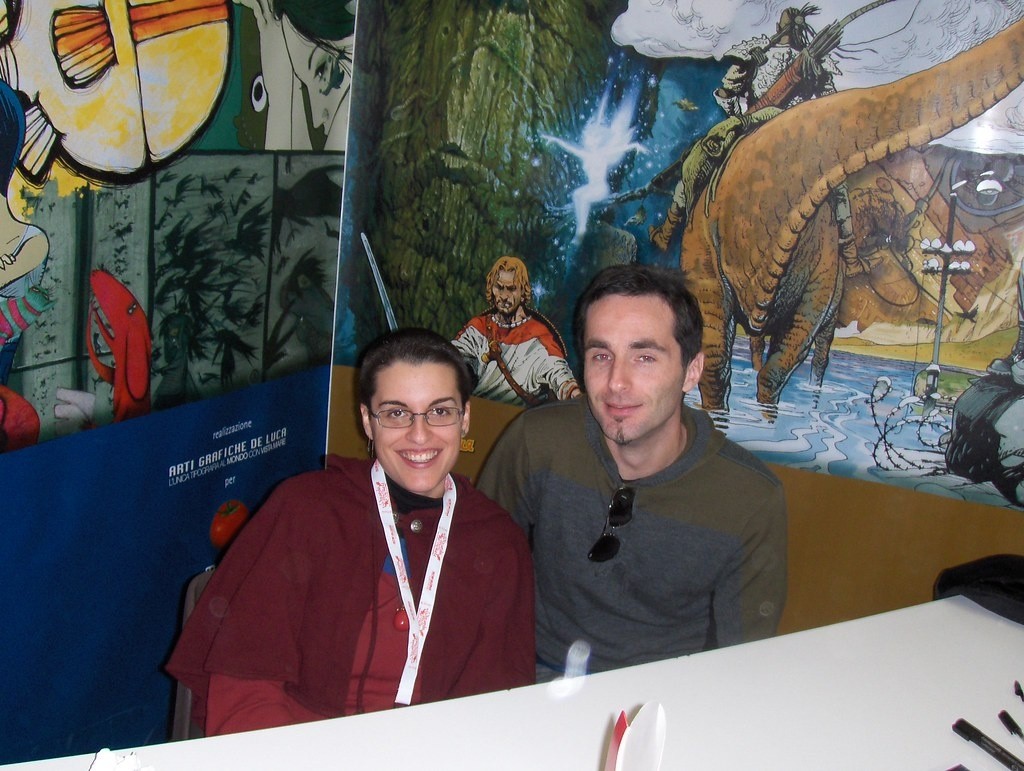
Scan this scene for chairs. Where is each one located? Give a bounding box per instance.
[172,569,218,741]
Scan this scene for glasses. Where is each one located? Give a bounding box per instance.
[369,407,465,429]
[587,482,635,563]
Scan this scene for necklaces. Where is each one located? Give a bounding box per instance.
[388,550,410,632]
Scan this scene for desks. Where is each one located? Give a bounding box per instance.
[0,553,1024,771]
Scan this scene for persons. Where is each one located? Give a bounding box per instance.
[478,262,786,685]
[167,327,537,736]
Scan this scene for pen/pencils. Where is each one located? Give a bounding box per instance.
[997,709,1024,741]
[1013,680,1024,701]
[951,718,1024,771]
